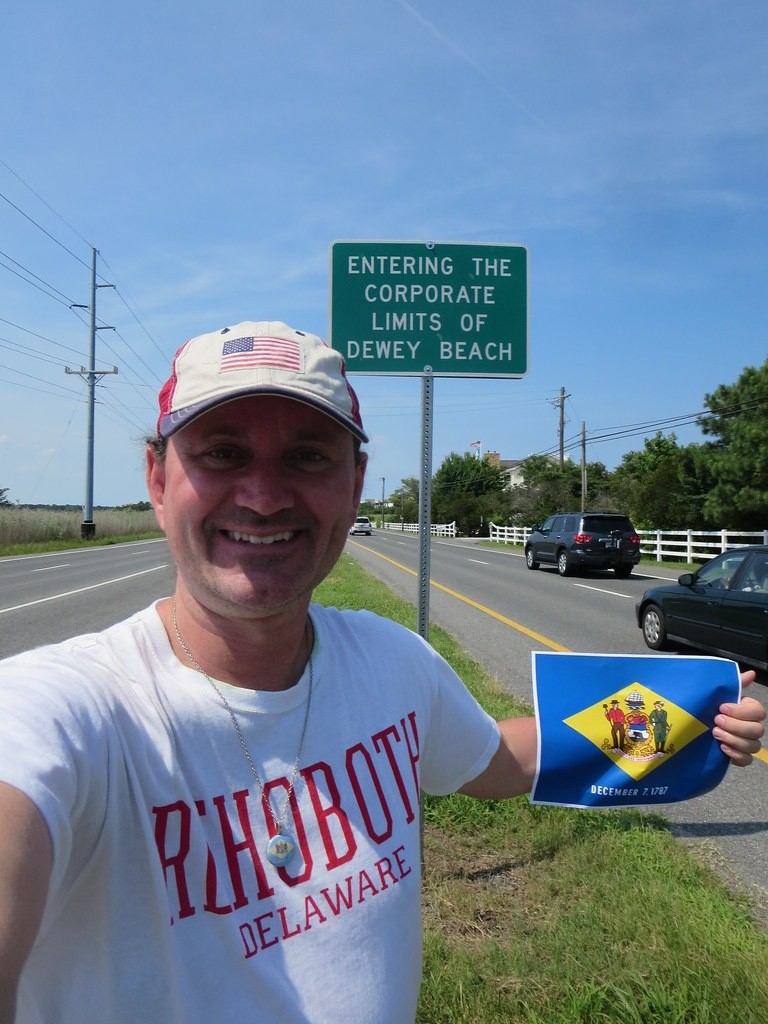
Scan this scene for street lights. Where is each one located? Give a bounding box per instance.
[380,477,386,529]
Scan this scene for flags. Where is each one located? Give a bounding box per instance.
[471,440,480,447]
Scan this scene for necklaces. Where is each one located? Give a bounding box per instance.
[173,599,313,867]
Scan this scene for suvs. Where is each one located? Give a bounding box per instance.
[524,511,641,578]
[349,516,372,536]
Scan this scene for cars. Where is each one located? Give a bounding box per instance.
[636,544,768,675]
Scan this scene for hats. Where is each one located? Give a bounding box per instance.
[156,321,370,444]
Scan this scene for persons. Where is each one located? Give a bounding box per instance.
[0,321,768,1024]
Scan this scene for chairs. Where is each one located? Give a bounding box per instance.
[753,573,768,593]
